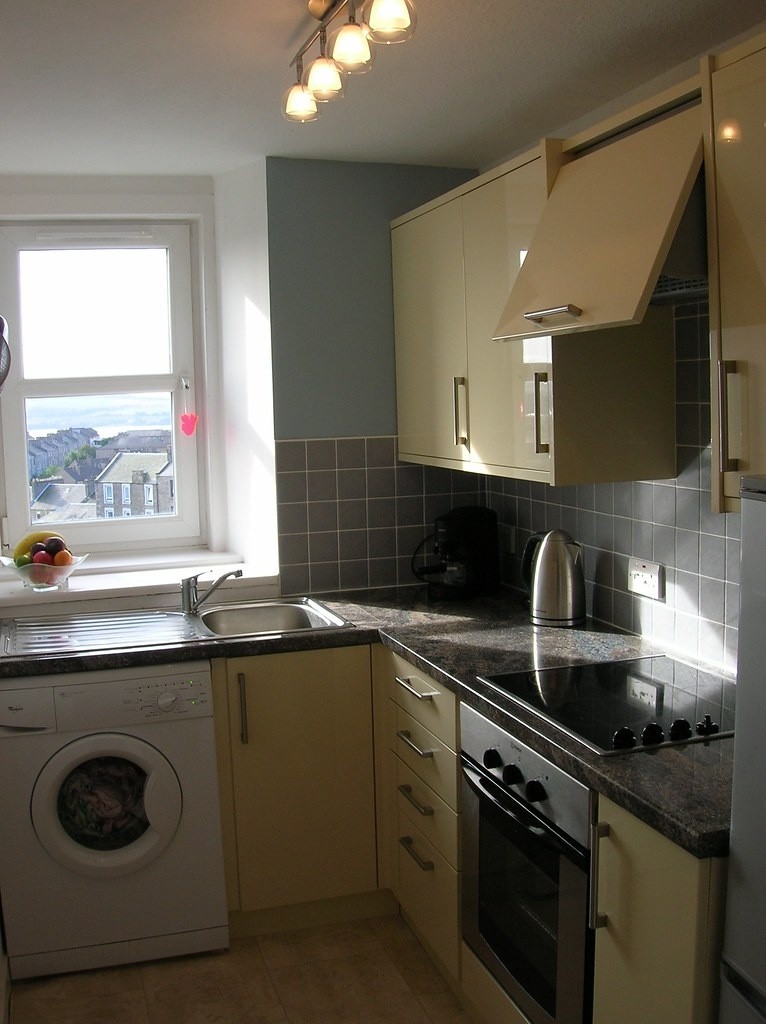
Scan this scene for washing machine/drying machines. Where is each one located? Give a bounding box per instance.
[0,659,232,982]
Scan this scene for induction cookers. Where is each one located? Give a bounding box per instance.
[460,654,733,760]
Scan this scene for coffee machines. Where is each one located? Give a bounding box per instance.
[411,506,500,600]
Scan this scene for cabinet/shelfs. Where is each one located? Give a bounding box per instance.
[387,30,766,514]
[212,642,728,1024]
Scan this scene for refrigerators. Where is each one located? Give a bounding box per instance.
[719,475,766,1024]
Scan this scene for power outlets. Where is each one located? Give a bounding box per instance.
[626,674,664,717]
[497,525,516,553]
[628,558,666,600]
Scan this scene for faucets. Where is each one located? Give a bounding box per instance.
[180,569,243,615]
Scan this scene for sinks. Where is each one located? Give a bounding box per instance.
[197,595,356,639]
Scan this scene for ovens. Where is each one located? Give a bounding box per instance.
[458,700,593,1024]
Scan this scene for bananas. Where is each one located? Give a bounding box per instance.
[13,530,73,563]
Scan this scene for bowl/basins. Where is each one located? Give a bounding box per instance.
[0,553,89,591]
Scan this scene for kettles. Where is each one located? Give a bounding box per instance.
[521,531,590,627]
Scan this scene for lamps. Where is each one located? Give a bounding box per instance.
[281,56,322,123]
[301,27,347,103]
[325,0,376,75]
[359,0,418,44]
